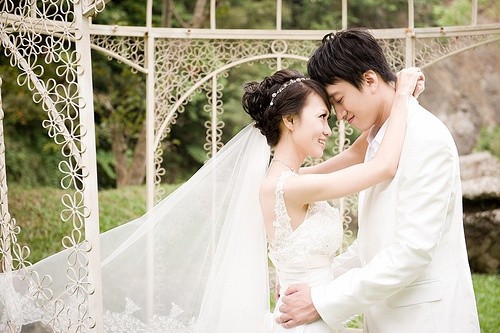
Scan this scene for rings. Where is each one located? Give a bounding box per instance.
[416,67,421,72]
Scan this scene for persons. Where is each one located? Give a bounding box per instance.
[242,68,425,332]
[275,29,481,332]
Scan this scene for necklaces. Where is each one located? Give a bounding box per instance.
[272,157,295,173]
